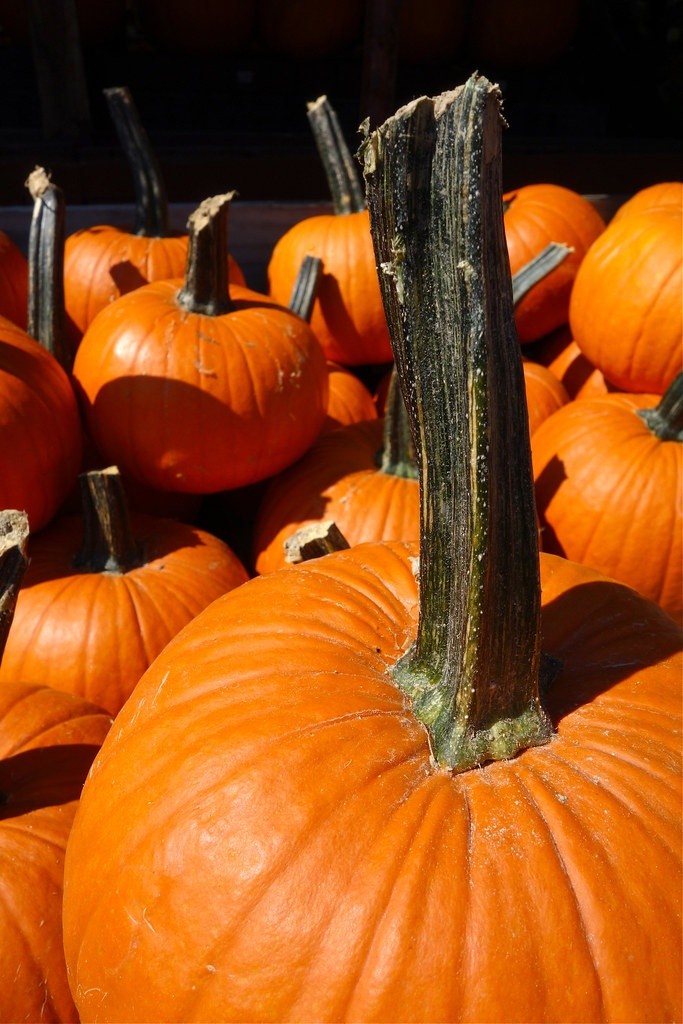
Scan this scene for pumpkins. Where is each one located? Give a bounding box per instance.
[0,83,683,1024]
